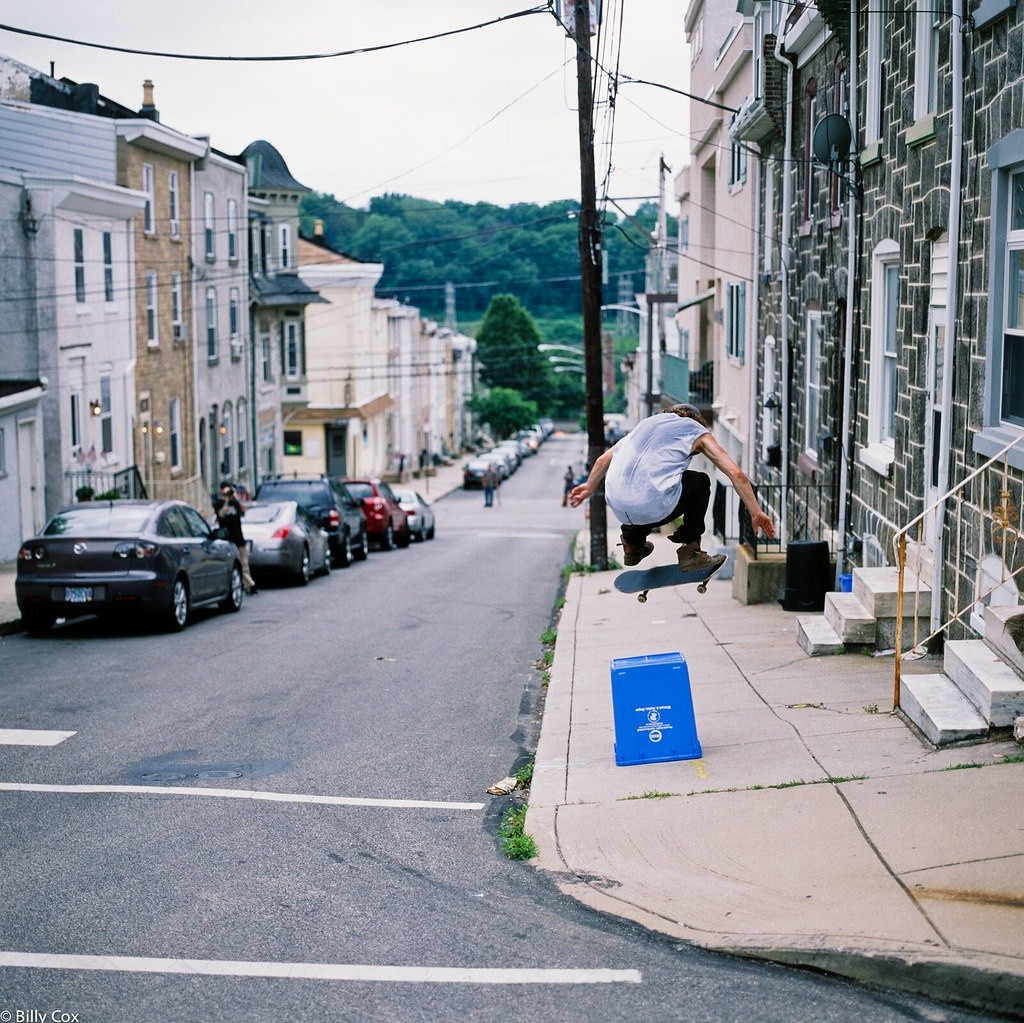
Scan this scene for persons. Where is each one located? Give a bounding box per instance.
[212,482,258,596]
[482,464,499,507]
[568,404,775,573]
[561,466,574,506]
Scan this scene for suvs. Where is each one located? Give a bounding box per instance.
[252,471,370,566]
[332,474,415,551]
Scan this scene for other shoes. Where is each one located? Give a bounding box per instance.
[676,541,725,572]
[615,534,655,565]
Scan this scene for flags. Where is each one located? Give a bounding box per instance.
[76,447,107,465]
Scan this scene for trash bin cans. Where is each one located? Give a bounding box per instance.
[609,650,703,767]
[777,538,834,613]
[839,573,853,592]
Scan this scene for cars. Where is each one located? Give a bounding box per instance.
[389,485,437,543]
[460,418,555,490]
[14,497,243,634]
[206,498,333,586]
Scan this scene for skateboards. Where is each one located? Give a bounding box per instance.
[614,554,728,603]
